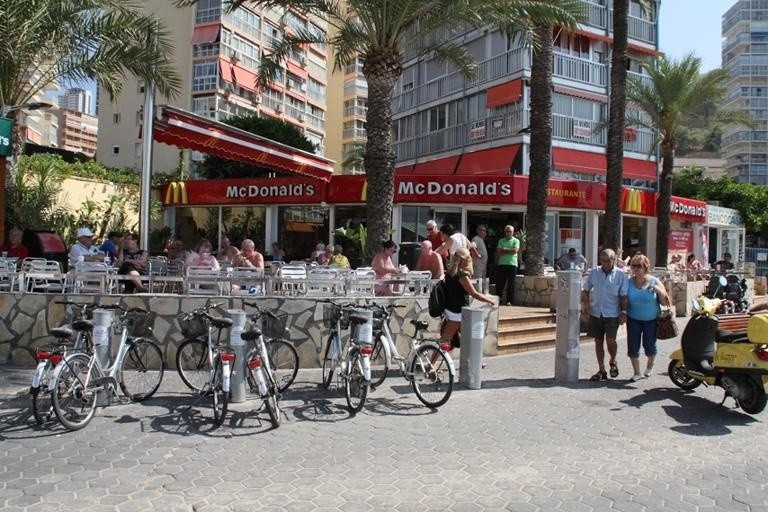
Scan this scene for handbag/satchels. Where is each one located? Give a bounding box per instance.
[428,280,446,317]
[656,295,679,340]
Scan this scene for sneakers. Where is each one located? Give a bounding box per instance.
[644,367,652,377]
[631,374,640,381]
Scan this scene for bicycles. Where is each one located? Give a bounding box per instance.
[314,298,372,413]
[29,300,118,424]
[44,303,166,430]
[176,300,236,426]
[241,302,299,428]
[350,301,456,408]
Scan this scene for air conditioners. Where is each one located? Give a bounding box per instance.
[253,55,309,123]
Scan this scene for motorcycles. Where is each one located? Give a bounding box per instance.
[668,275,768,414]
[699,262,746,314]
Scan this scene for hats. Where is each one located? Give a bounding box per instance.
[76,227,93,238]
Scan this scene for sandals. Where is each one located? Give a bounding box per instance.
[591,369,607,381]
[610,360,618,377]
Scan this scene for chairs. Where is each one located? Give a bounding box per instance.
[0,241,484,303]
[556,258,751,289]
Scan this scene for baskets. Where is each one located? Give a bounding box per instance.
[369,305,393,330]
[126,311,156,337]
[176,309,207,336]
[262,308,288,337]
[71,303,94,321]
[323,304,351,329]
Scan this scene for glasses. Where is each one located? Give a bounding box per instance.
[426,226,435,231]
[631,265,642,268]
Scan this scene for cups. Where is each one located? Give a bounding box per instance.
[77,256,84,262]
[104,256,109,263]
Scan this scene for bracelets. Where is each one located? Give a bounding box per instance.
[620,308,627,314]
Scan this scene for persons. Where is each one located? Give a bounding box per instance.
[0,227,29,272]
[553,248,588,274]
[494,224,521,307]
[414,220,495,343]
[68,233,149,295]
[618,251,671,383]
[581,248,629,381]
[163,236,287,276]
[371,239,402,295]
[311,242,352,269]
[665,252,737,282]
